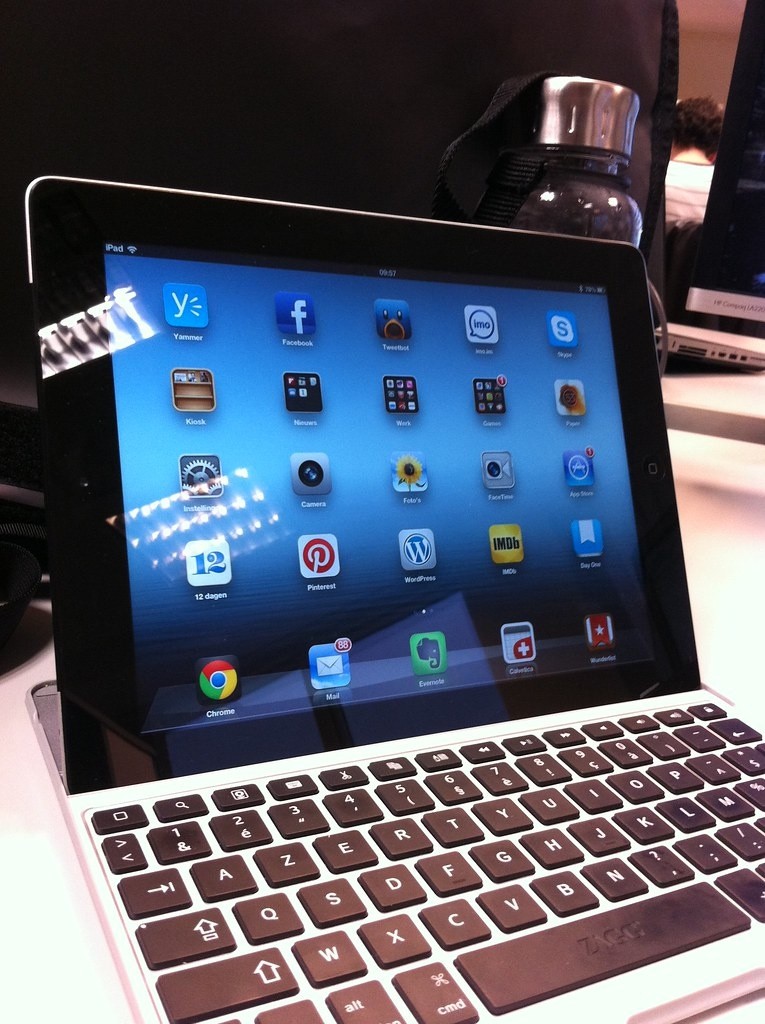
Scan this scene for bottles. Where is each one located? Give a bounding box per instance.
[470,76,643,249]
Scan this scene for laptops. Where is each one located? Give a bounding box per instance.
[22,177,765,1024]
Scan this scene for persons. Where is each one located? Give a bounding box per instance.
[666,90,719,236]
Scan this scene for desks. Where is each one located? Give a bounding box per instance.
[0,371,765,1023]
